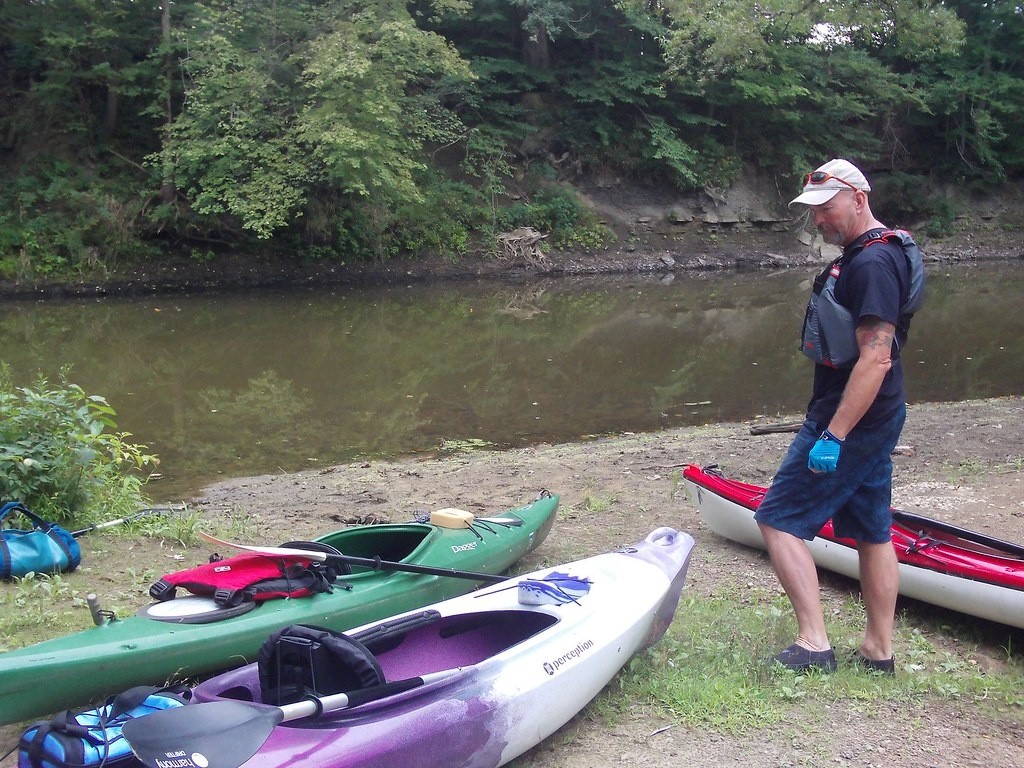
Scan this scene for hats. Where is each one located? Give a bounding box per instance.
[788,158,871,210]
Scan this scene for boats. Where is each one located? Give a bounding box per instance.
[15,528,696,768]
[683,463,1024,628]
[0,494,561,729]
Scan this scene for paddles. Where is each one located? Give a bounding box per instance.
[70,509,173,538]
[200,533,516,582]
[121,664,473,768]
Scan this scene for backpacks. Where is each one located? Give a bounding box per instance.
[149,543,354,601]
[18,684,189,768]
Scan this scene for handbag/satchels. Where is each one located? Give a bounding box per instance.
[0,497,82,582]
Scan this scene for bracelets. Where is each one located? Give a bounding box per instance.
[825,430,844,446]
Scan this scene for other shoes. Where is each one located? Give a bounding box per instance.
[767,643,837,674]
[838,648,895,679]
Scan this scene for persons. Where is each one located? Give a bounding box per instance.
[752,158,922,680]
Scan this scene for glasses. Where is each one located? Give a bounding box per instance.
[803,172,857,192]
